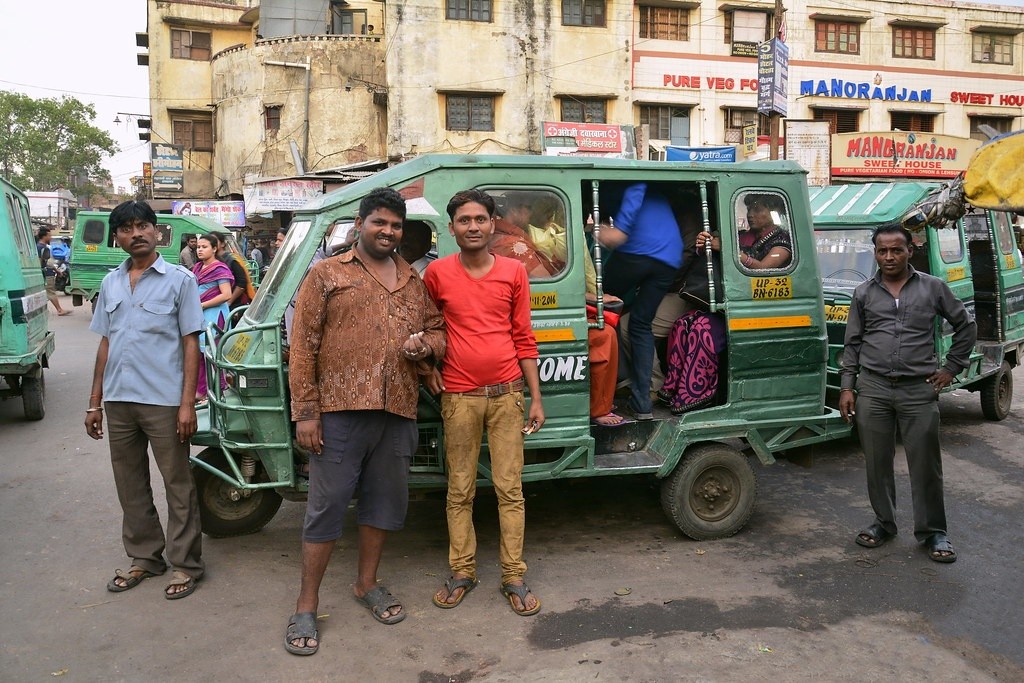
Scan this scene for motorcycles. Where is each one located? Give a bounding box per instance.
[185,156,855,545]
[64,208,262,316]
[805,178,1024,422]
[0,177,56,420]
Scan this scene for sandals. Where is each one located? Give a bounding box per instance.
[106,566,155,592]
[163,570,197,600]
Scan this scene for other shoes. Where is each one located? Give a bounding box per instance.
[58,309,73,317]
[619,401,654,420]
[194,399,209,410]
[616,378,632,390]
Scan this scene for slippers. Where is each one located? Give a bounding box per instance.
[592,412,623,427]
[499,581,541,616]
[611,404,618,411]
[923,533,957,562]
[351,586,406,626]
[855,523,890,548]
[284,612,320,656]
[431,575,477,609]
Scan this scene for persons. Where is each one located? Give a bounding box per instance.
[37,226,73,316]
[489,182,792,427]
[284,187,446,656]
[422,187,545,618]
[839,224,977,563]
[179,222,431,410]
[85,199,206,599]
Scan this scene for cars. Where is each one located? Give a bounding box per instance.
[46,235,73,267]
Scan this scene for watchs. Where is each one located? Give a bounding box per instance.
[202,304,205,310]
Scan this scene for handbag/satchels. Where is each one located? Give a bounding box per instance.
[676,235,760,321]
[585,292,624,329]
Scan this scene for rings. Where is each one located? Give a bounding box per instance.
[419,348,427,355]
[406,351,411,356]
[411,352,418,356]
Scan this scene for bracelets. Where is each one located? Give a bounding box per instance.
[86,407,104,412]
[90,395,103,399]
[744,252,753,268]
[840,389,854,393]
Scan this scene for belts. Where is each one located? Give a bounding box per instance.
[458,381,525,397]
